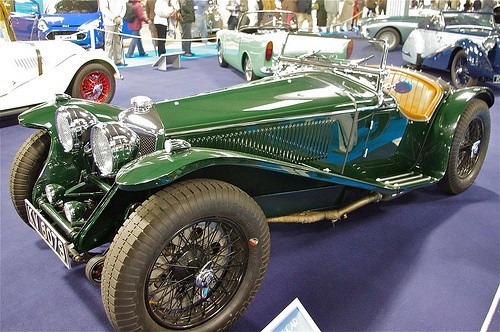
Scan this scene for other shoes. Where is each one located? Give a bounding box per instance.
[116,62,128,66]
[140,53,148,56]
[184,52,194,57]
[316,24,361,33]
[126,55,134,58]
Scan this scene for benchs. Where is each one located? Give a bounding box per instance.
[359,65,442,120]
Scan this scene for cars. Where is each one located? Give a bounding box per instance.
[360,13,443,53]
[12,56,494,328]
[402,12,499,89]
[217,11,353,80]
[10,1,104,50]
[0,40,122,119]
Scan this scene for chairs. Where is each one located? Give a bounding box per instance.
[73,1,91,11]
[55,0,72,11]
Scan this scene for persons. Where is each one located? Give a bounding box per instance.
[145,0,159,46]
[99,0,128,66]
[248,0,498,33]
[178,0,212,56]
[154,0,174,58]
[226,0,249,30]
[126,0,152,58]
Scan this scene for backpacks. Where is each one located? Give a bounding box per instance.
[124,2,136,22]
